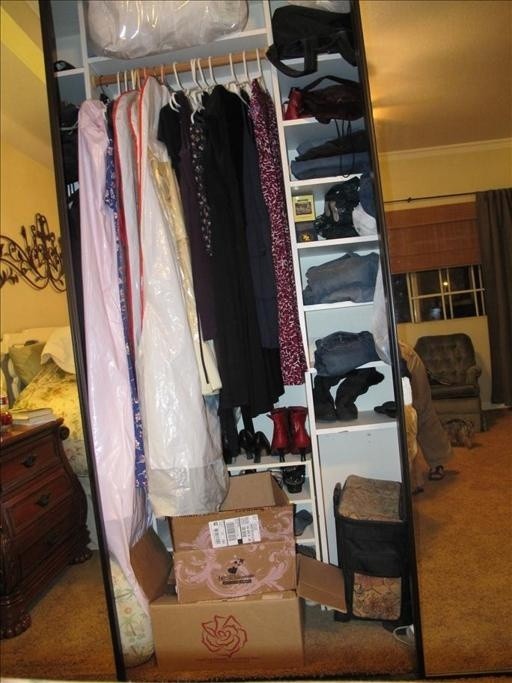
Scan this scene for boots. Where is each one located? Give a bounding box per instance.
[217,405,240,463]
[270,406,291,462]
[288,406,310,461]
[312,371,356,422]
[338,368,384,421]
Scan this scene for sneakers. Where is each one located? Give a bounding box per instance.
[296,542,316,558]
[294,509,313,537]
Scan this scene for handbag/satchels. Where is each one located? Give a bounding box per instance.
[302,83,363,121]
[284,88,313,120]
[267,5,356,77]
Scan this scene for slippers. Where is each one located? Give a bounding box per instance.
[429,464,445,480]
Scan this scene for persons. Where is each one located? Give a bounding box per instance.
[397,340,455,481]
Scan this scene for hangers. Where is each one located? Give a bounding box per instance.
[79,48,273,126]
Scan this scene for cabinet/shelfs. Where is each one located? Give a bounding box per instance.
[0,417,92,638]
[38,0,424,683]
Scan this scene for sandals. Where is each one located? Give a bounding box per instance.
[267,468,283,490]
[283,467,306,493]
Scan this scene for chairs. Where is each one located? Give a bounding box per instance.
[414,334,482,434]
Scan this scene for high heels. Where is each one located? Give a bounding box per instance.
[252,432,270,463]
[238,429,253,459]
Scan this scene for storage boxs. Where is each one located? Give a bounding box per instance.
[129,503,347,671]
[217,473,290,510]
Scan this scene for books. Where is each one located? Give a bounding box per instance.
[9,408,53,420]
